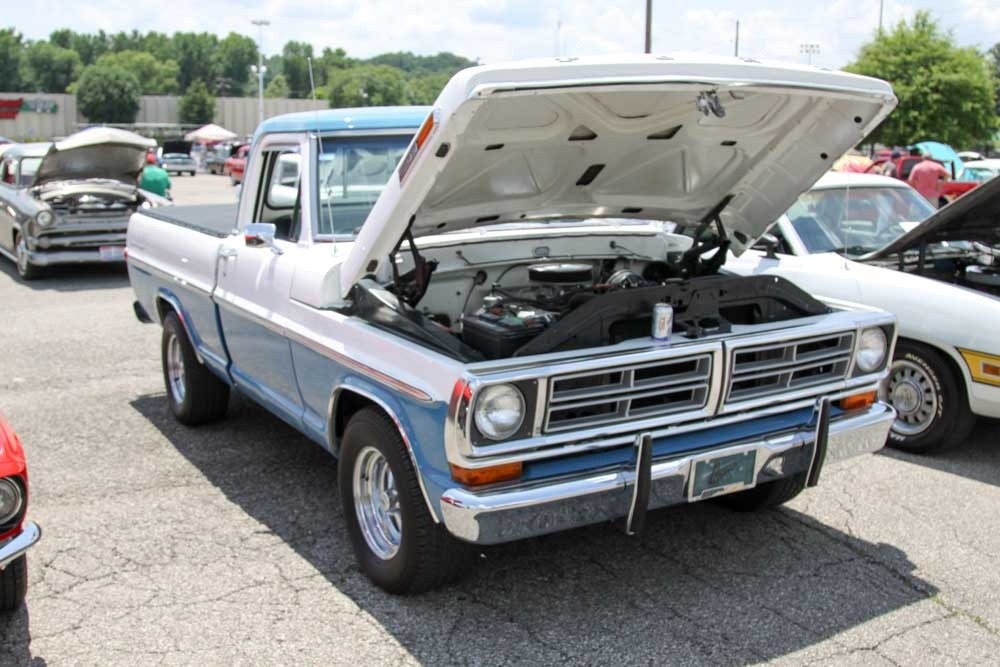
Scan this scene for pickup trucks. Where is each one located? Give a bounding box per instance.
[123,53,900,601]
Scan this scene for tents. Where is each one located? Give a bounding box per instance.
[184,123,237,141]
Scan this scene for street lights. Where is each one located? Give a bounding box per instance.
[251,19,272,121]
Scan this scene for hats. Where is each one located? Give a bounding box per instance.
[147,153,154,163]
[890,152,902,159]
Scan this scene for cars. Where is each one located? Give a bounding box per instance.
[0,125,175,282]
[203,141,387,210]
[146,137,197,177]
[671,169,1000,458]
[810,146,1000,229]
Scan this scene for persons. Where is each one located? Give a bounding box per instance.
[188,140,233,172]
[875,147,949,234]
[137,152,170,200]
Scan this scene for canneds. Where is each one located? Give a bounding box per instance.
[651,302,674,342]
